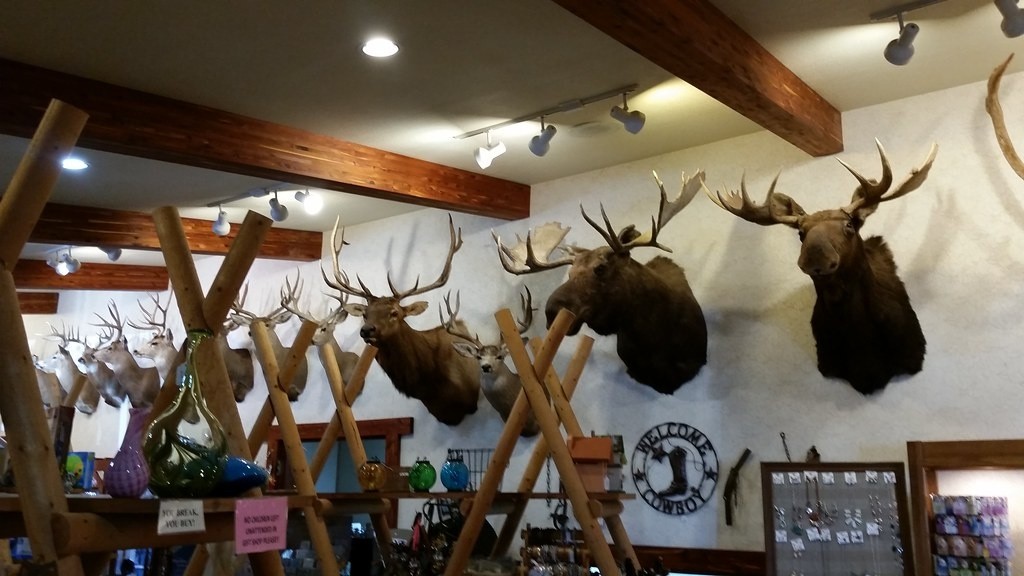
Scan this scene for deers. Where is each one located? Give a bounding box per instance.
[12,52,1024,438]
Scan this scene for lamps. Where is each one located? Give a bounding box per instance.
[474,132,505,171]
[528,116,557,156]
[994,0,1024,38]
[884,10,919,65]
[46,252,69,276]
[212,204,231,235]
[269,190,289,222]
[611,93,646,134]
[295,189,323,214]
[98,246,122,261]
[62,246,81,274]
